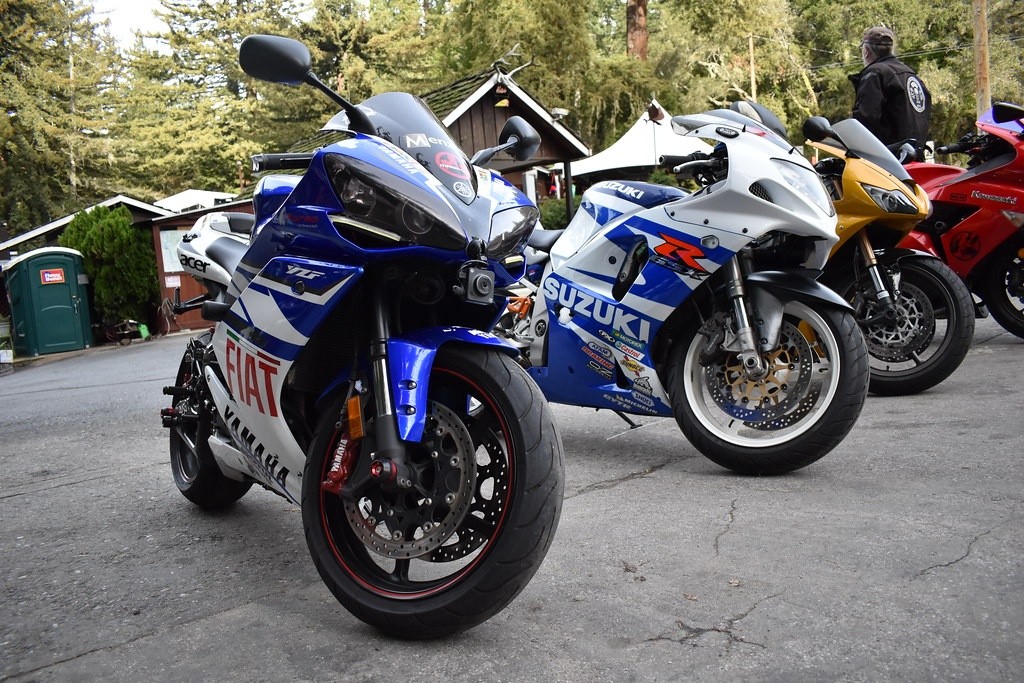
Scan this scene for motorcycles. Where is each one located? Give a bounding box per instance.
[902,98,1024,342]
[490,96,870,475]
[791,113,974,397]
[155,29,565,643]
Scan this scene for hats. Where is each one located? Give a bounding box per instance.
[858,26,894,49]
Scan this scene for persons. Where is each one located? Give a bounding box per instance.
[847,26,932,165]
